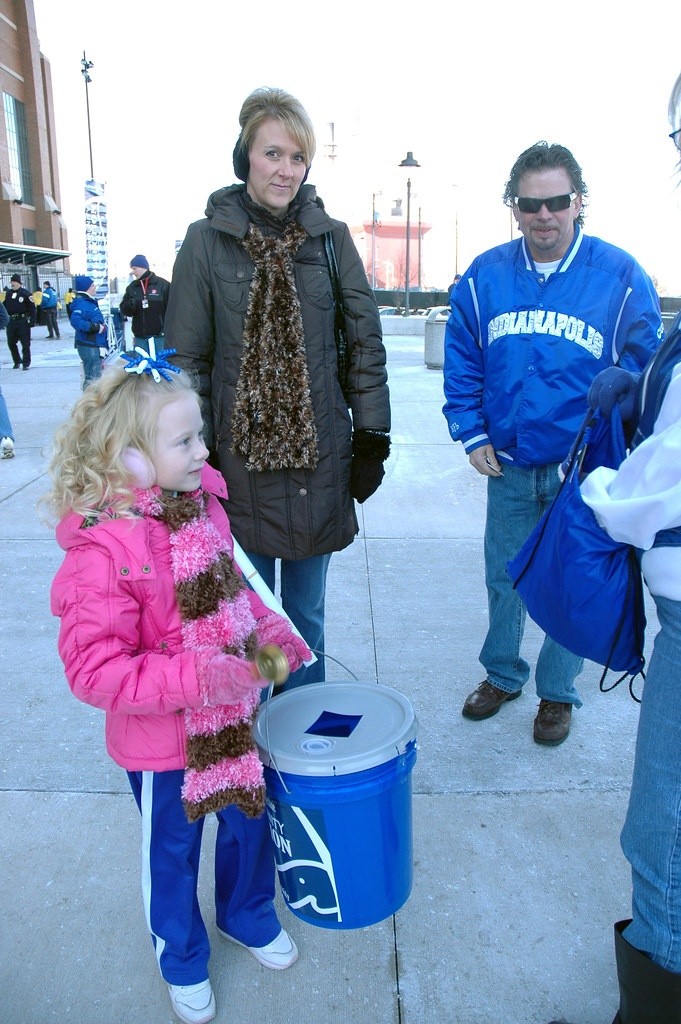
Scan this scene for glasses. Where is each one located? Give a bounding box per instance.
[514,192,578,213]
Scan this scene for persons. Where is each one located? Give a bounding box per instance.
[166,87,392,709]
[49,348,316,1023]
[70,275,110,394]
[0,299,16,461]
[0,273,76,372]
[579,80,680,1024]
[441,143,667,749]
[121,254,171,361]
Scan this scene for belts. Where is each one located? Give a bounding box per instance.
[9,312,30,318]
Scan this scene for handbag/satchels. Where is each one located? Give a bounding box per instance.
[507,402,646,676]
[334,305,352,409]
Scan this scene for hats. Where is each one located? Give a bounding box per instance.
[75,276,94,292]
[11,274,21,283]
[131,255,149,269]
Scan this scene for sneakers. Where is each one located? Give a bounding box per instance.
[0,437,15,459]
[215,924,298,971]
[168,979,216,1024]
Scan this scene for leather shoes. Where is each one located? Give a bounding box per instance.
[534,699,572,746]
[462,679,523,720]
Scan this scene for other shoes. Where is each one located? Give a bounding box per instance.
[13,359,21,369]
[23,366,29,370]
[46,335,54,338]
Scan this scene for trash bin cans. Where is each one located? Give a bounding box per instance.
[96,308,127,358]
[423,304,451,369]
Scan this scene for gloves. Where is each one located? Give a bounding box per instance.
[255,614,312,672]
[587,366,643,421]
[351,430,392,504]
[196,645,269,706]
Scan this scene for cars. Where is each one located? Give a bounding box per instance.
[377,306,449,316]
[658,296,681,333]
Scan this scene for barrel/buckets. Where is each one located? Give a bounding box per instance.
[252,646,419,929]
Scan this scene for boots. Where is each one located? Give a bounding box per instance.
[547,918,681,1024]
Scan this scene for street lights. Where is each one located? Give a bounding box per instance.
[82,59,95,175]
[398,152,422,314]
